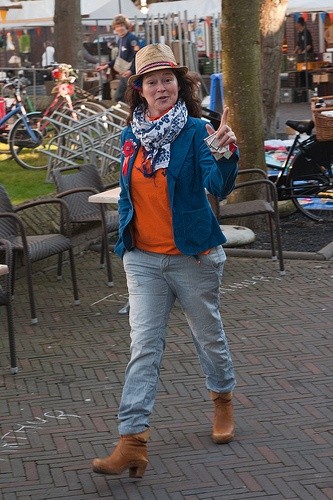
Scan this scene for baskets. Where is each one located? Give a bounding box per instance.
[311,95,333,142]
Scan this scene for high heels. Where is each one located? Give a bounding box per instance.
[92,429,149,478]
[210,391,235,443]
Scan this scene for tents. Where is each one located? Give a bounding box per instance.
[0,0,333,101]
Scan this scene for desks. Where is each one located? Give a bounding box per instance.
[88,187,209,203]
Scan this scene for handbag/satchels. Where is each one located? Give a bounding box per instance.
[113,56,131,75]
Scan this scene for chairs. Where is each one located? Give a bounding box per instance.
[206,168,286,276]
[51,163,120,287]
[0,185,80,324]
[0,239,18,375]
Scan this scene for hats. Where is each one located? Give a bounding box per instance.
[128,43,189,85]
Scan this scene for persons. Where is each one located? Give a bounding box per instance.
[92,43,239,478]
[41,41,55,81]
[106,40,119,81]
[292,17,314,86]
[95,15,141,102]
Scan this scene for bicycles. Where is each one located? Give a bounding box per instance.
[0,60,116,172]
[197,104,333,224]
